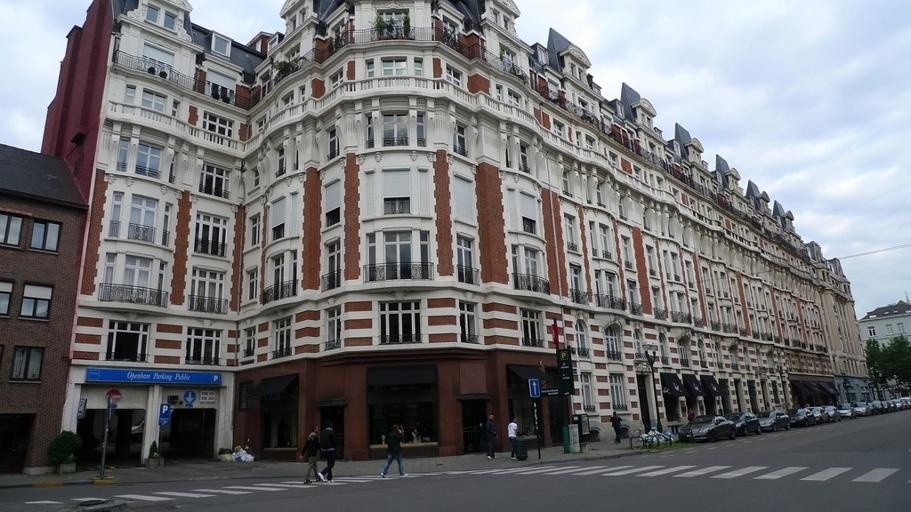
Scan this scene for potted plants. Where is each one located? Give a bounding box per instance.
[144,441,160,467]
[217,448,234,461]
[47,431,82,474]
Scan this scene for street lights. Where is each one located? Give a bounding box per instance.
[774,359,790,412]
[642,340,663,432]
[839,367,852,404]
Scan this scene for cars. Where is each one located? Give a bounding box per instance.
[788,396,910,428]
[680,414,736,442]
[757,409,791,431]
[725,412,762,436]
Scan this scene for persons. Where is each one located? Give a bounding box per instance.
[485,413,499,460]
[317,420,338,482]
[507,416,518,459]
[300,424,321,484]
[610,412,622,443]
[688,407,695,421]
[381,424,408,478]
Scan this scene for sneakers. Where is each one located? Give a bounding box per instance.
[399,473,409,478]
[381,472,388,478]
[304,478,334,484]
[317,472,327,482]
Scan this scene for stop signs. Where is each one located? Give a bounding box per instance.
[105,389,121,404]
